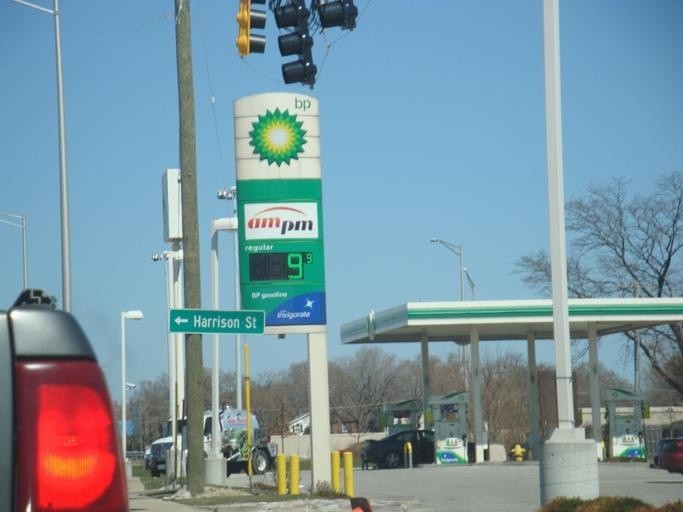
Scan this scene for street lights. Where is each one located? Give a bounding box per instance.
[119,308,143,461]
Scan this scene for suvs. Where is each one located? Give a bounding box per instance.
[144,409,276,478]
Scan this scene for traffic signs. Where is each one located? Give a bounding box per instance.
[167,308,268,338]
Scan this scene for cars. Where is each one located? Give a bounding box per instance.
[359,429,487,470]
